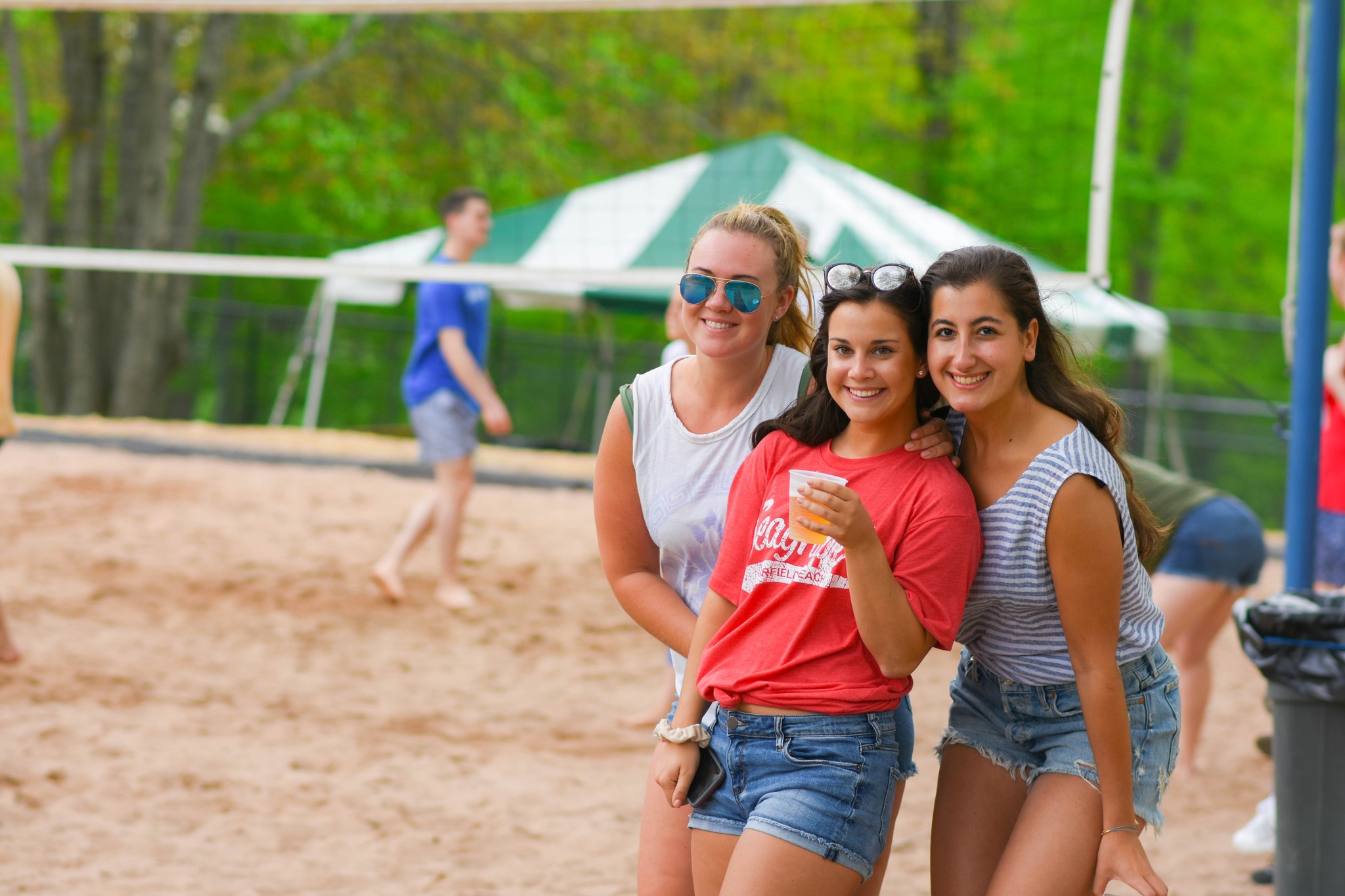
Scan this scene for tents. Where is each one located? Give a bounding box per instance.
[265,131,1189,476]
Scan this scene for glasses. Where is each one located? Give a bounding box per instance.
[824,262,923,315]
[679,273,779,313]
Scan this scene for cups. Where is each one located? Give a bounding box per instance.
[788,469,848,547]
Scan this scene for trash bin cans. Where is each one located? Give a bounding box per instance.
[1233,587,1345,896]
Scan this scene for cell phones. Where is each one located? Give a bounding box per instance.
[686,746,727,808]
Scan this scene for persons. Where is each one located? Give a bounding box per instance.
[1230,219,1345,854]
[1120,451,1267,776]
[649,263,984,896]
[0,258,22,664]
[370,186,515,611]
[920,244,1181,896]
[594,193,961,896]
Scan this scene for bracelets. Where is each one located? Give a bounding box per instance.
[1101,825,1139,835]
[653,718,711,749]
